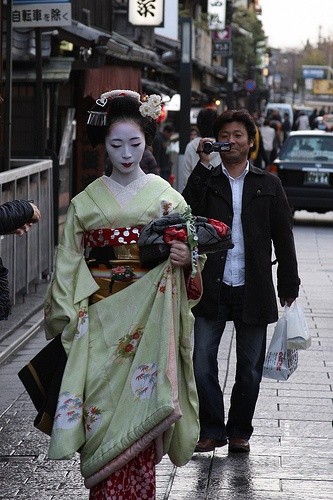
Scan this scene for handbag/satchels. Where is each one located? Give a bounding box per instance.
[263,301,298,381]
[136,208,232,263]
[286,296,312,351]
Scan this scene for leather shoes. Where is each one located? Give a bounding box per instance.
[228,436,250,452]
[194,433,227,451]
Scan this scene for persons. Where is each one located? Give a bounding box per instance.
[0,200,42,321]
[40,88,207,500]
[140,103,333,179]
[182,108,300,452]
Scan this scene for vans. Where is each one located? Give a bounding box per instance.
[263,102,294,130]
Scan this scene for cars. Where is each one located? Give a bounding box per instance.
[295,108,313,116]
[269,130,333,216]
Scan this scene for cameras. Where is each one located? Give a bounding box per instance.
[202,141,231,154]
